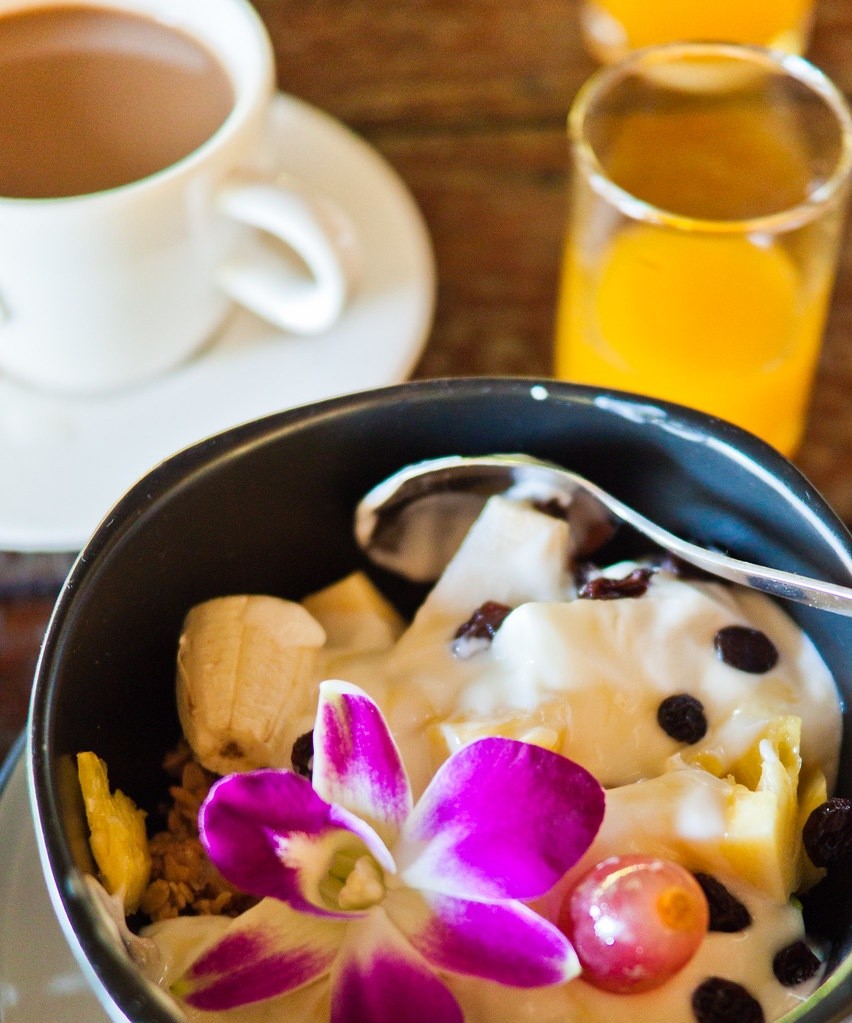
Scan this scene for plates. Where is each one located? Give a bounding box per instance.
[3,724,111,1023]
[2,87,440,560]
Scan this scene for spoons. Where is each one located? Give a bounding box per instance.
[351,446,852,622]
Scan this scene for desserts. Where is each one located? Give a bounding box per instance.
[83,449,849,1019]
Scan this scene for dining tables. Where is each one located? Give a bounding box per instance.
[0,0,852,773]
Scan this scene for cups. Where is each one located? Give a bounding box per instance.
[555,37,850,463]
[0,0,354,392]
[571,0,817,97]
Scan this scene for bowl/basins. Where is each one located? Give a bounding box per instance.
[26,367,852,1023]
